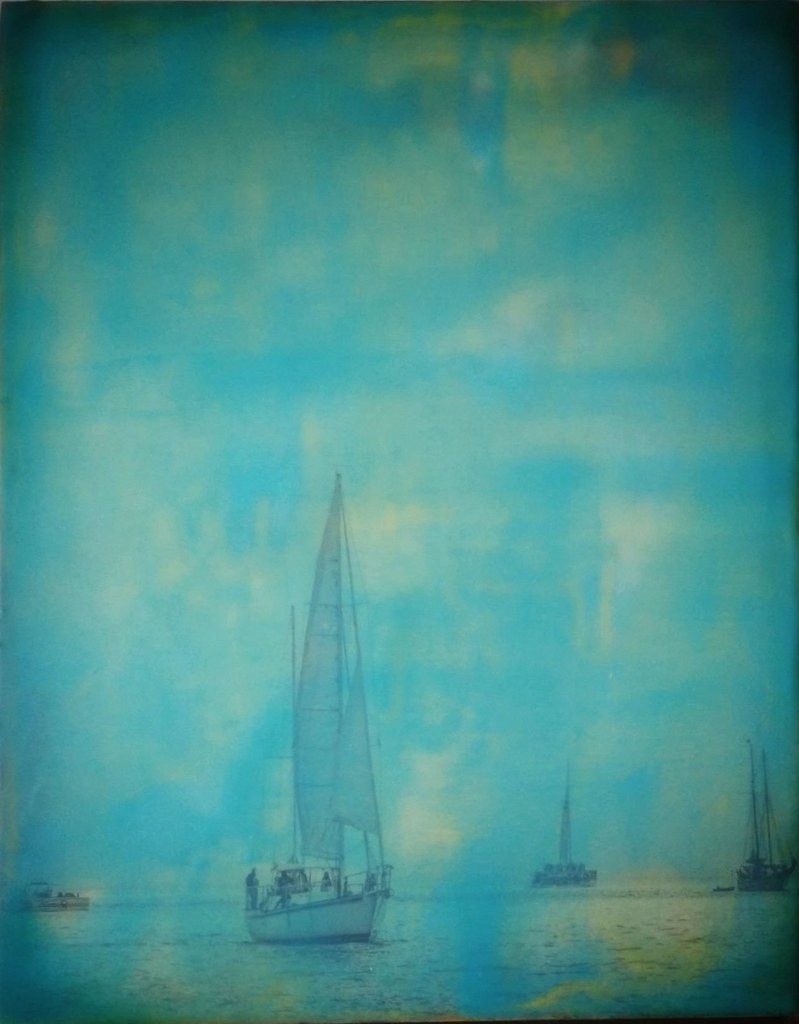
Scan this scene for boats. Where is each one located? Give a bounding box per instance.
[713,885,735,892]
[13,880,91,912]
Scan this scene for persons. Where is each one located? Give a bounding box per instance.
[320,872,331,892]
[246,866,259,908]
[279,871,308,895]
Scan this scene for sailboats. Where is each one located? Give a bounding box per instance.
[530,767,598,889]
[735,737,797,892]
[242,469,394,946]
